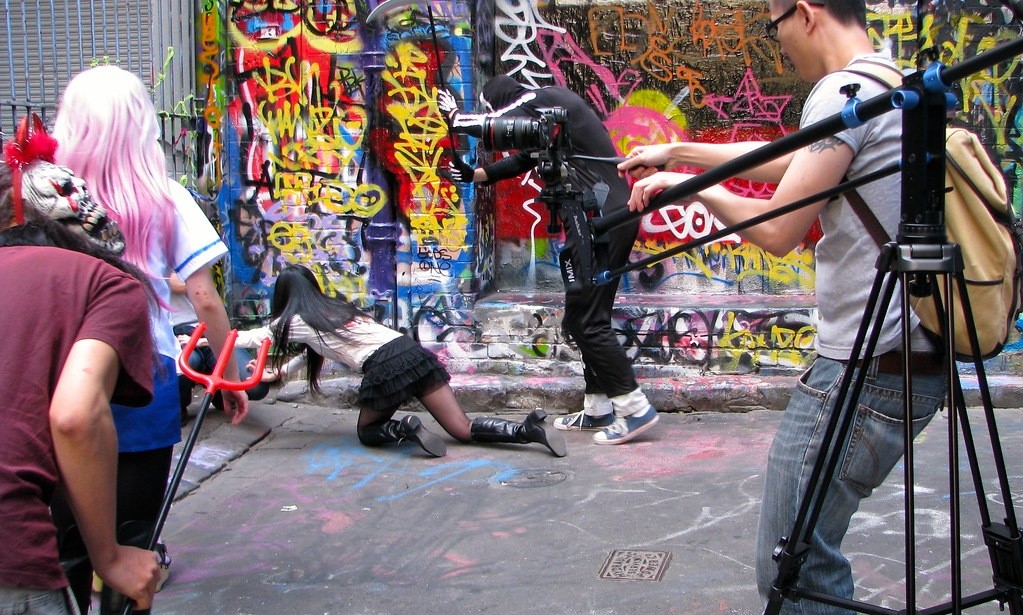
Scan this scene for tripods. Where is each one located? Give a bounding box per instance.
[560,37,1023,615]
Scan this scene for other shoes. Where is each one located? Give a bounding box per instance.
[211,388,238,410]
[180,405,189,428]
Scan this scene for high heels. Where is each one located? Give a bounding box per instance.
[153,542,172,592]
[92,569,103,592]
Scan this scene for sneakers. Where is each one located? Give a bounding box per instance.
[592,403,661,445]
[554,410,615,431]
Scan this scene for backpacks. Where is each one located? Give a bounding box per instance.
[840,60,1023,363]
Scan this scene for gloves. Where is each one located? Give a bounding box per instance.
[436,87,459,120]
[448,153,474,183]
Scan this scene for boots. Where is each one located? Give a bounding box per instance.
[470,407,567,457]
[359,414,447,457]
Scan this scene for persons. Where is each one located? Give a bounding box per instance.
[167,269,269,427]
[435,74,660,446]
[0,159,163,614]
[175,264,567,457]
[52,65,249,614]
[618,0,954,615]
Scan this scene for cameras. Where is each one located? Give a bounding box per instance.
[481,102,586,235]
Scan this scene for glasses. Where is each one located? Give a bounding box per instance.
[765,2,825,43]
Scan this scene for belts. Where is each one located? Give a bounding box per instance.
[840,350,950,375]
